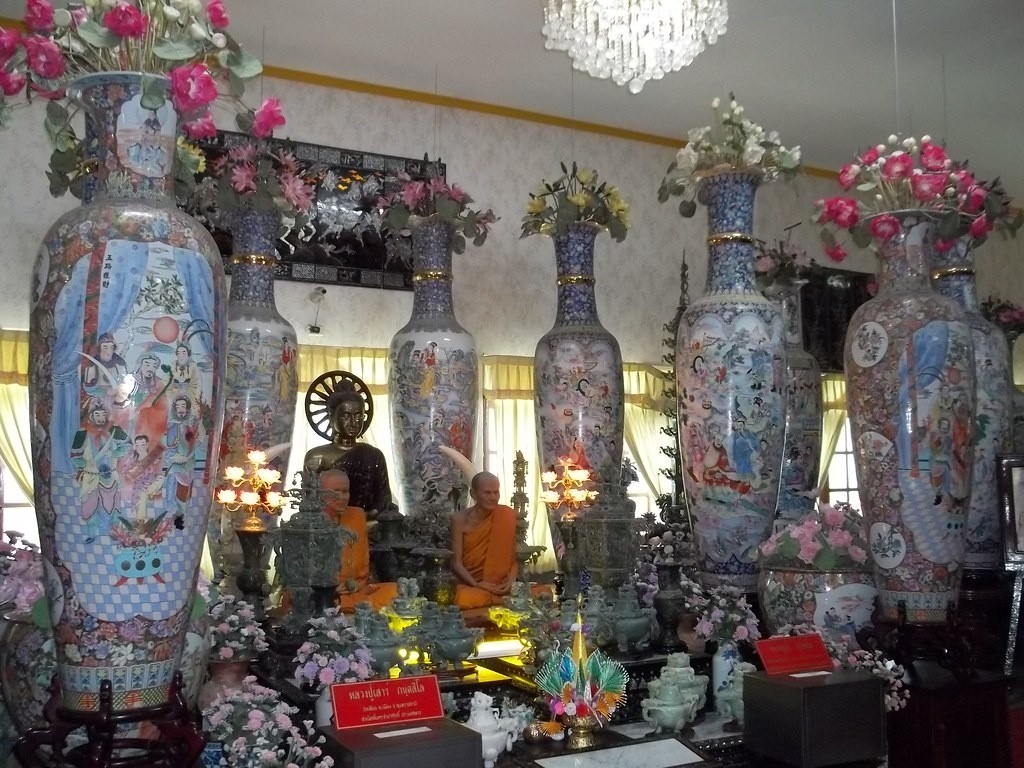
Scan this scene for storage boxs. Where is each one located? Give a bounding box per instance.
[316,716,482,768]
[743,667,889,768]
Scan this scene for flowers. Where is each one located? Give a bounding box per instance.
[518,161,631,244]
[758,487,870,571]
[752,237,816,297]
[0,0,287,206]
[207,596,270,660]
[656,90,801,218]
[372,152,502,268]
[769,623,911,712]
[292,604,378,691]
[202,675,334,768]
[688,585,762,642]
[633,513,699,606]
[0,529,219,630]
[189,142,323,239]
[979,295,1024,336]
[805,131,1024,264]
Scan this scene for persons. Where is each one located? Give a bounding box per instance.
[452,471,553,609]
[301,378,392,586]
[320,469,398,613]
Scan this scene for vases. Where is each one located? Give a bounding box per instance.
[757,570,878,635]
[235,530,267,602]
[757,278,822,494]
[531,219,625,592]
[205,205,301,602]
[842,207,978,623]
[314,684,334,727]
[654,561,686,648]
[675,169,788,591]
[387,225,478,526]
[929,268,1012,571]
[311,580,341,620]
[712,640,740,712]
[196,661,250,741]
[6,614,204,734]
[28,72,227,761]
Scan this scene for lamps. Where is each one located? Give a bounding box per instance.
[216,449,288,531]
[541,0,730,95]
[538,456,599,521]
